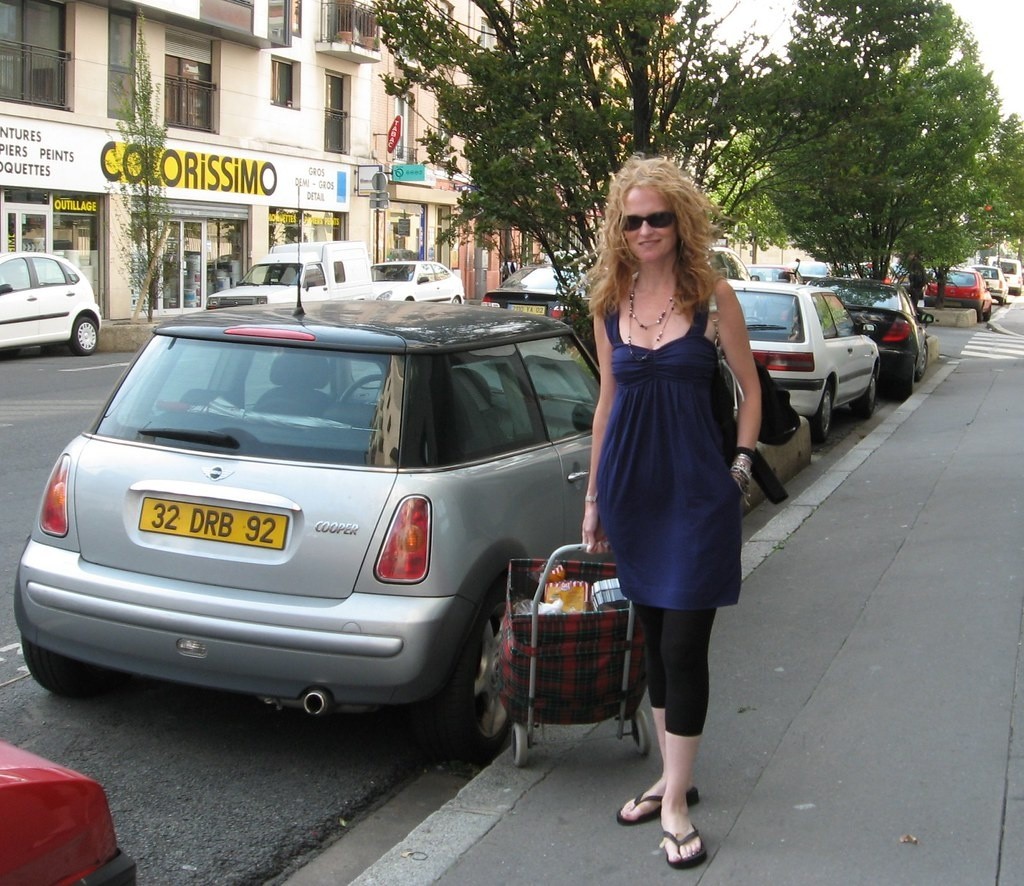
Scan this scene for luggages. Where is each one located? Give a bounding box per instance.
[499,542,651,765]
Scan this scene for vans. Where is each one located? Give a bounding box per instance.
[987,258,1024,296]
[536,249,597,271]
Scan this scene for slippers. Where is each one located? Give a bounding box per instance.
[616,786,699,826]
[663,825,707,870]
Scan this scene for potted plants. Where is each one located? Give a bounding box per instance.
[354,4,378,49]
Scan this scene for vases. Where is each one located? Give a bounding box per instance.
[336,30,352,44]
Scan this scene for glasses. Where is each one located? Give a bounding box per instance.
[619,210,677,231]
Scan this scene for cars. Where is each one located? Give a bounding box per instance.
[965,264,1011,306]
[15,299,617,766]
[707,246,912,293]
[708,278,882,444]
[370,260,466,304]
[0,738,137,886]
[923,267,994,323]
[479,263,607,326]
[0,251,102,356]
[791,277,931,398]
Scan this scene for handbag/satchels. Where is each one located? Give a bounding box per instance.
[708,276,801,446]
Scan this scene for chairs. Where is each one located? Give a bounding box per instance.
[255,355,330,420]
[433,367,516,453]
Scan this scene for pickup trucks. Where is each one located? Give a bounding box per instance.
[205,241,372,311]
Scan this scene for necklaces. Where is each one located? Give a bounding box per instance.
[626,274,676,363]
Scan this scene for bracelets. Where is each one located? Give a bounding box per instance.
[584,496,596,502]
[730,447,755,503]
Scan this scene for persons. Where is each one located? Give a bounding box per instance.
[581,154,763,868]
[499,254,516,282]
[751,275,760,281]
